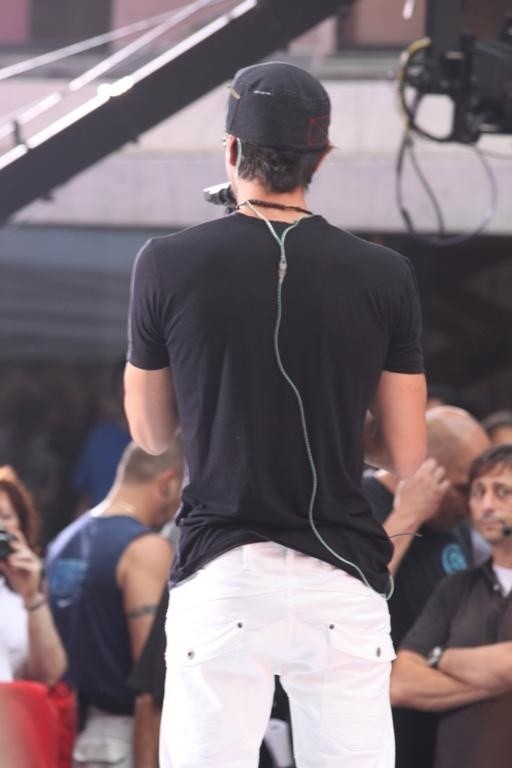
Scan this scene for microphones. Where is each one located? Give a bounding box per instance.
[501,525,512,534]
[204,184,235,204]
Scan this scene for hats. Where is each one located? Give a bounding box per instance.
[226,62,329,149]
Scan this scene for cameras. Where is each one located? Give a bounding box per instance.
[1,529,15,558]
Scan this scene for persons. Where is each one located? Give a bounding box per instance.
[123,60,425,768]
[0,382,511,768]
